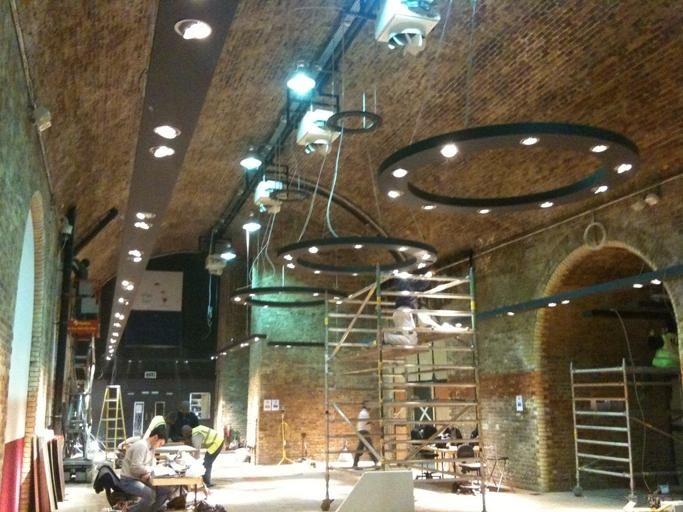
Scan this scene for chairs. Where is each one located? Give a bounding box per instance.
[453,445,486,496]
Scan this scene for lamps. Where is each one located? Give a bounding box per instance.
[220,240,252,262]
[284,58,341,91]
[240,138,290,171]
[241,206,271,232]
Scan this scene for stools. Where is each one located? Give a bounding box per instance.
[415,452,442,481]
[102,479,138,509]
[487,455,514,493]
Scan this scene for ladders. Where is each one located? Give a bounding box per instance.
[95,385,127,460]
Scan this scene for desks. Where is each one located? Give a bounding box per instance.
[152,477,202,505]
[415,446,483,479]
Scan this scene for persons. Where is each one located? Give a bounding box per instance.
[180,423,225,488]
[380,268,437,346]
[351,396,382,471]
[169,400,200,442]
[117,424,174,511]
